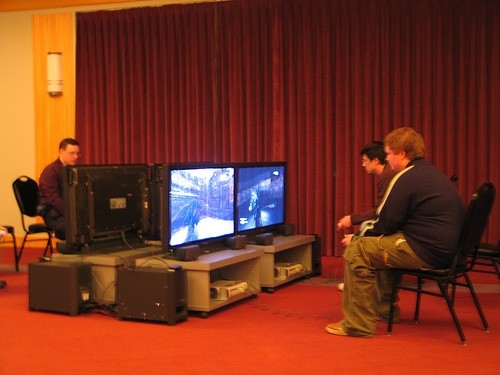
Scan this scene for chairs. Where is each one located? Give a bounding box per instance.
[12,176,58,270]
[386,182,497,342]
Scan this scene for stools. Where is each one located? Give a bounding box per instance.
[0,224,20,273]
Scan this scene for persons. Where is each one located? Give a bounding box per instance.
[37,138,81,240]
[325,126,467,337]
[337,141,398,291]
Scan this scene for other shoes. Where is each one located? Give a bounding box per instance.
[338,282,345,291]
[326,322,350,335]
[375,303,401,322]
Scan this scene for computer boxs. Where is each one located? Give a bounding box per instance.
[115,266,187,324]
[28,259,93,316]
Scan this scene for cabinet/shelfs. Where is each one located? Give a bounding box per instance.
[51,231,321,319]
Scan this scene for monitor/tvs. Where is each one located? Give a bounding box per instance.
[62,161,287,253]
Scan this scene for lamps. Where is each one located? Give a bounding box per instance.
[46,52,63,97]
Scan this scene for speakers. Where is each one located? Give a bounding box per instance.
[227,235,247,250]
[175,245,200,261]
[279,223,295,236]
[255,233,274,245]
